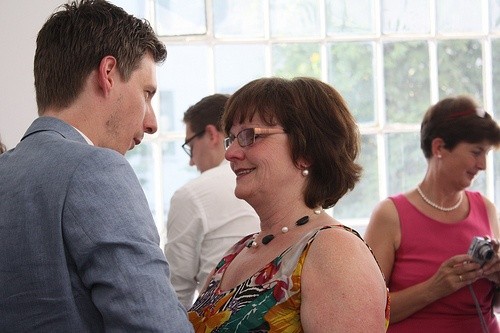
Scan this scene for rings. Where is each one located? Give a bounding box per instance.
[460,275,463,280]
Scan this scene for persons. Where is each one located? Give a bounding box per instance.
[187,77,391,333]
[165,94,260,309]
[0,0,196,333]
[363,95,500,333]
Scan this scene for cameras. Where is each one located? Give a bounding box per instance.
[463,237,494,268]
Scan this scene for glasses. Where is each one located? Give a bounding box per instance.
[224,127,290,152]
[183,128,207,158]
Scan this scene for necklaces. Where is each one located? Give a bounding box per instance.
[246,207,323,248]
[416,184,463,211]
